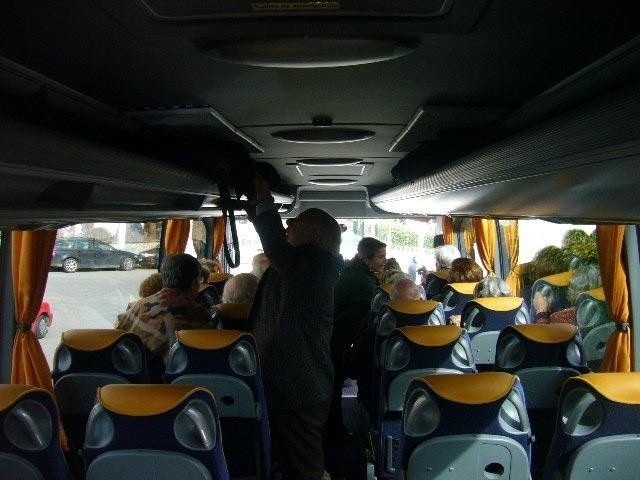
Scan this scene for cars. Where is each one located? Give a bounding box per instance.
[30,300,53,339]
[48,237,137,273]
[137,247,161,269]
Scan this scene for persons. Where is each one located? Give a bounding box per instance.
[246,169,341,480]
[115,248,273,366]
[380,233,529,333]
[323,235,385,479]
[530,229,603,326]
